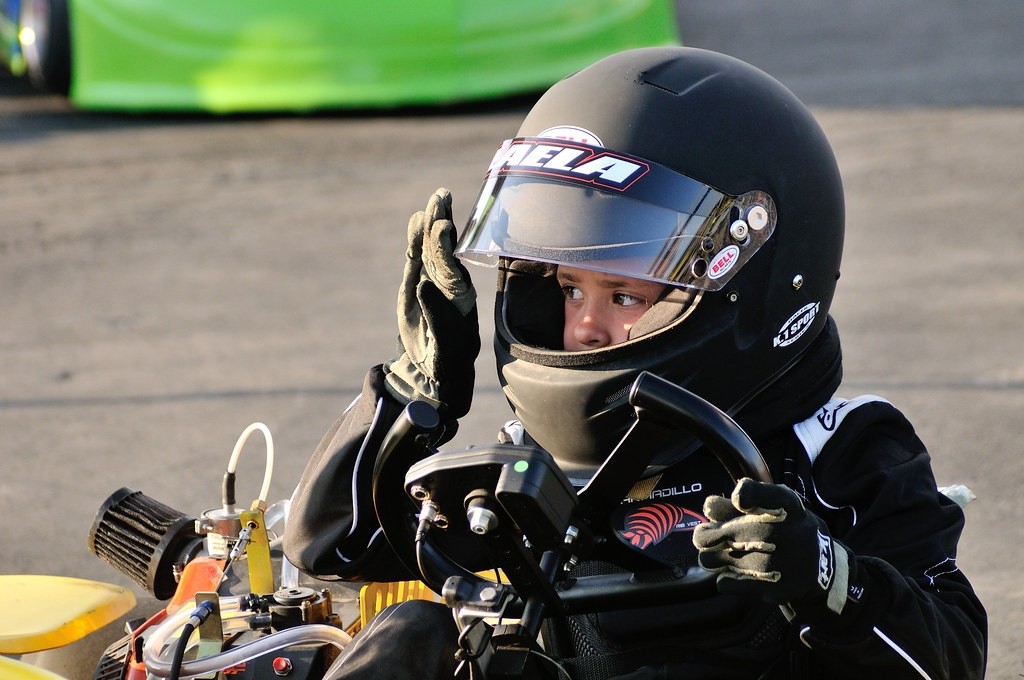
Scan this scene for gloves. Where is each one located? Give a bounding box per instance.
[377,185,482,423]
[693,476,850,626]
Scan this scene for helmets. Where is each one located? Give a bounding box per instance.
[450,45,845,487]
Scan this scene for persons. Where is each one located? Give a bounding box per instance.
[286,47,988,680]
